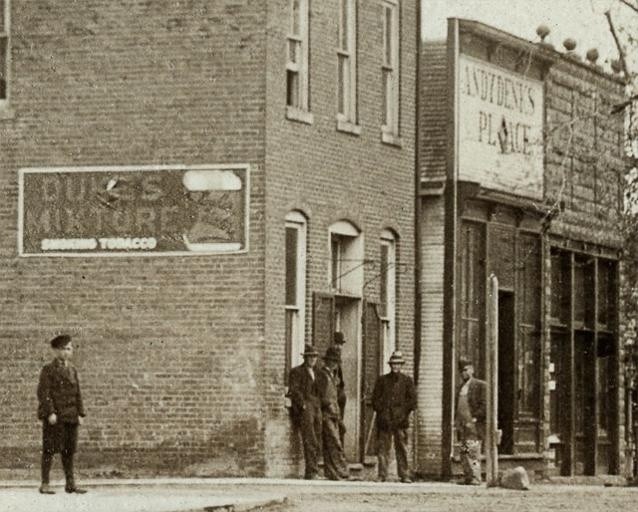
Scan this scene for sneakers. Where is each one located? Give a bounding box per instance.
[65,484,86,494]
[39,483,56,494]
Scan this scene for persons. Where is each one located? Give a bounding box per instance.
[288,332,363,481]
[35,333,87,495]
[449,355,490,485]
[372,351,417,484]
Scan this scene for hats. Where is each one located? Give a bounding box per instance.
[300,344,319,357]
[334,331,346,344]
[322,346,343,363]
[387,351,406,363]
[458,361,472,369]
[50,335,71,348]
